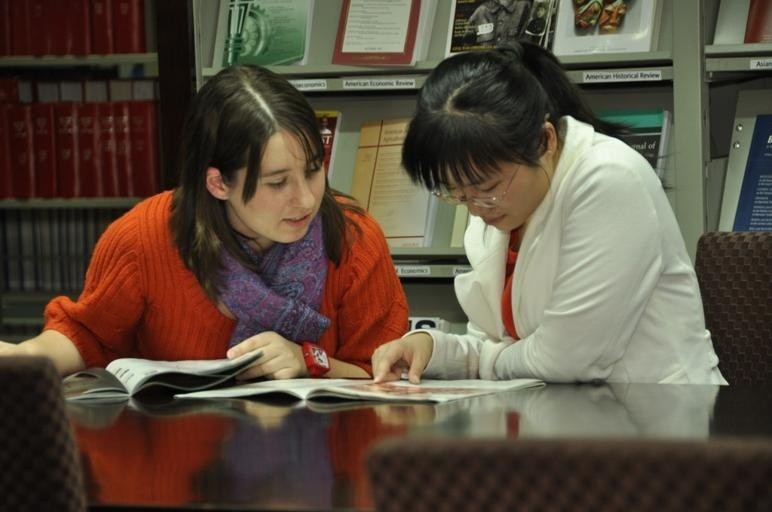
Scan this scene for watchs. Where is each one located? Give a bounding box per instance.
[302,341,331,378]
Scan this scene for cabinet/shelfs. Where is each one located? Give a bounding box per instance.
[1,0,200,330]
[193,0,772,324]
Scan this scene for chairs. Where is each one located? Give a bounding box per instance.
[372,436,772,512]
[693,227,772,395]
[0,354,94,510]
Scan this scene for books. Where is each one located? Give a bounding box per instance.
[443,0,560,63]
[713,0,772,47]
[331,0,440,67]
[173,378,547,404]
[450,203,469,248]
[313,109,343,185]
[64,348,265,399]
[551,0,663,55]
[717,88,771,232]
[352,116,441,250]
[4,209,114,292]
[0,0,149,57]
[578,105,671,192]
[407,316,468,335]
[212,0,315,70]
[0,79,157,198]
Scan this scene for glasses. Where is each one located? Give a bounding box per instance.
[428,110,554,206]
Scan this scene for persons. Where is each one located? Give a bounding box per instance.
[523,3,547,41]
[371,40,711,386]
[0,63,410,386]
